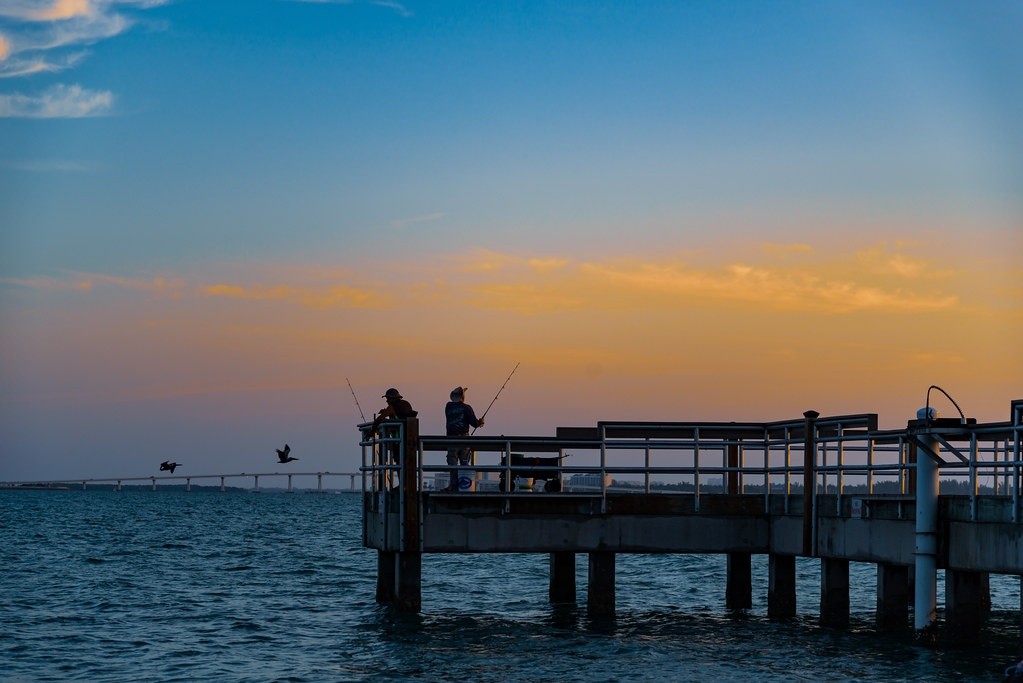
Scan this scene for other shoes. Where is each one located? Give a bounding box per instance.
[440,485,458,493]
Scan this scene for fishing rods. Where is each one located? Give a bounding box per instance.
[470,362,520,436]
[346,378,366,423]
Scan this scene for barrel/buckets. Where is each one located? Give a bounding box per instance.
[519,477,533,492]
[458,470,476,492]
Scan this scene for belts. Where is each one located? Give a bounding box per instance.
[455,431,468,435]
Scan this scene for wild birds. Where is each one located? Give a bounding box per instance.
[160,460,183,474]
[275,444,299,463]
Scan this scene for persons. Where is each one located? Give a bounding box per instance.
[372,388,418,490]
[439,386,484,492]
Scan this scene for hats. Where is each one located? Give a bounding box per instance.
[382,388,403,398]
[453,387,468,396]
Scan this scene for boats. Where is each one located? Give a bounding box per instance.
[1002,655,1023,683]
